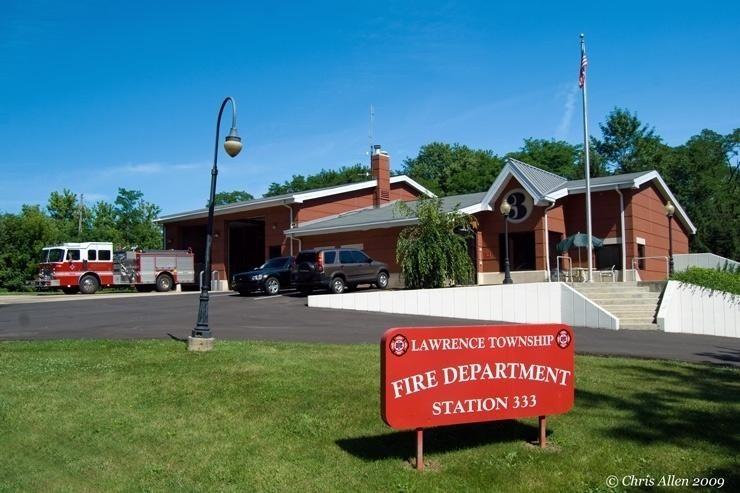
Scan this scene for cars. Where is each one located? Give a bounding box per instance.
[232,256,297,295]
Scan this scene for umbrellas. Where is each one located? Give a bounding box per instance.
[556,232,603,268]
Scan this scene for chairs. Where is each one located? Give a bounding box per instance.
[600,263,615,281]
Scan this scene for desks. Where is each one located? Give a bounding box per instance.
[569,267,597,282]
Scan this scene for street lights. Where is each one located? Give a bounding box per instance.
[499,199,513,284]
[663,201,675,277]
[187,95,243,354]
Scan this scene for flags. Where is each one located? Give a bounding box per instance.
[578,43,588,87]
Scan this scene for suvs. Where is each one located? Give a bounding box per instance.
[290,248,390,295]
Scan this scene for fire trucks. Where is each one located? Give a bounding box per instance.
[35,241,196,295]
